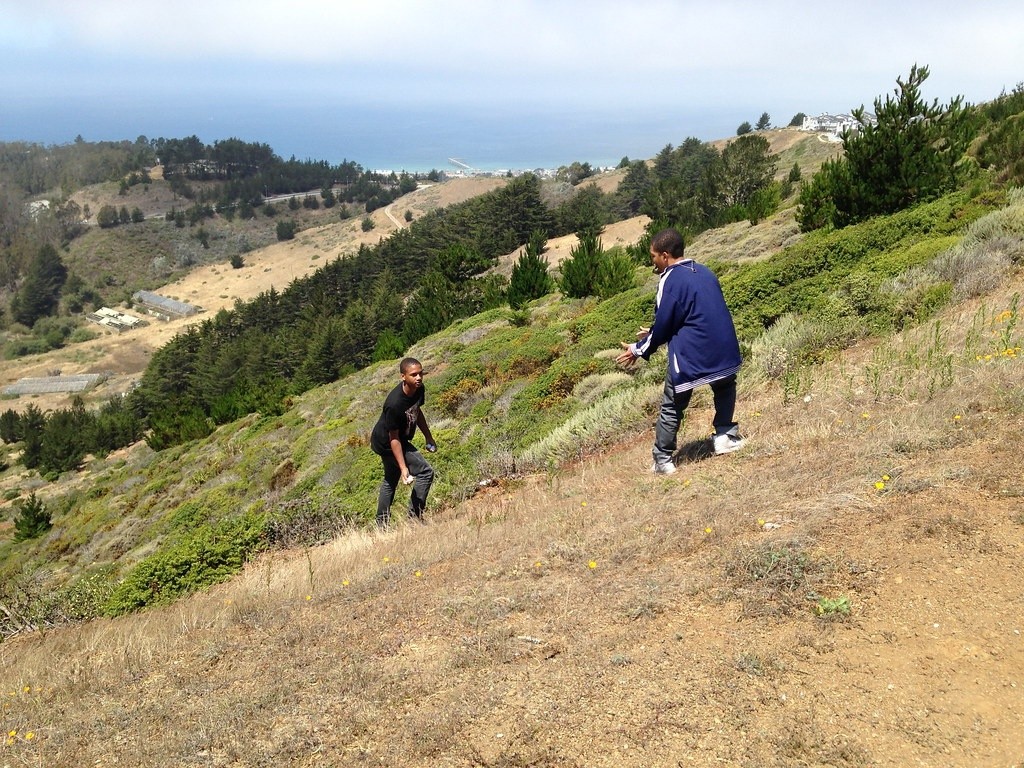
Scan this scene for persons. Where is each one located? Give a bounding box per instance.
[616,229,748,472]
[370,357,437,531]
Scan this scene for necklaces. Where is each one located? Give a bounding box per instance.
[675,260,697,273]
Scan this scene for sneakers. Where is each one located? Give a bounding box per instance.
[650,460,677,476]
[711,433,748,456]
